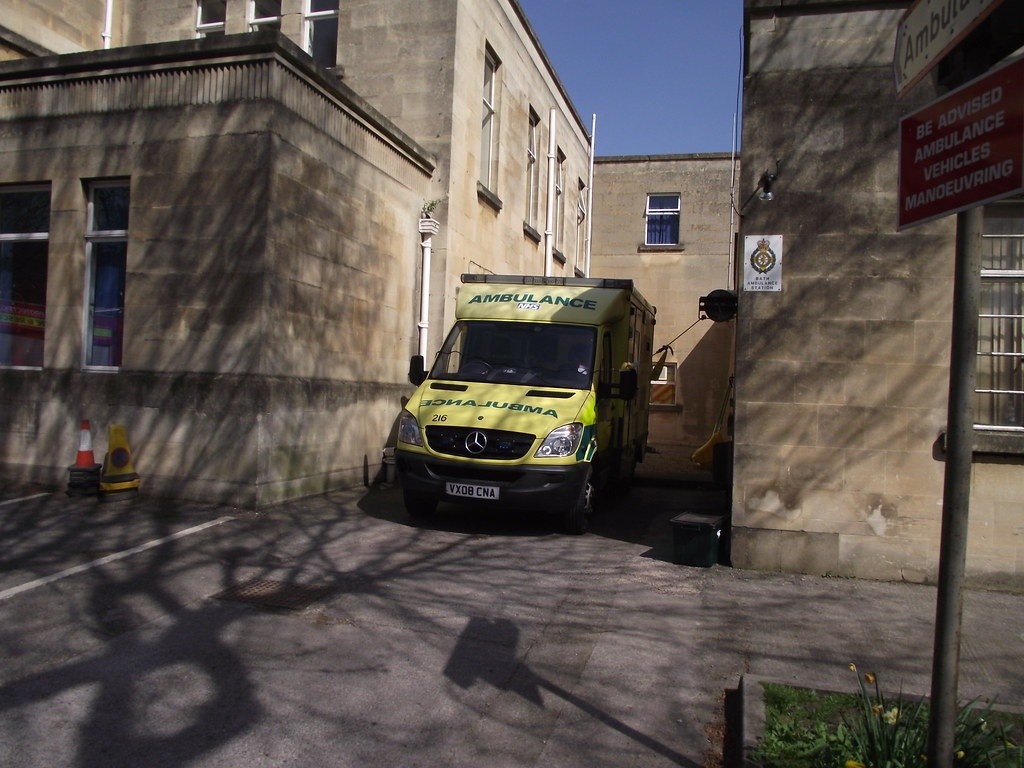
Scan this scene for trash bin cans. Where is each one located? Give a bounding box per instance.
[669,508,729,567]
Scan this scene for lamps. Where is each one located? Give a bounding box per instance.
[741,176,774,211]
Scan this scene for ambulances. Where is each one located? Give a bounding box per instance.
[395,272,657,526]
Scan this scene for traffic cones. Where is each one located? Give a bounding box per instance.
[65,418,103,496]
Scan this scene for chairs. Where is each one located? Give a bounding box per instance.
[477,337,521,379]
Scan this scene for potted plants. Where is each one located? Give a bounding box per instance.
[419,196,450,236]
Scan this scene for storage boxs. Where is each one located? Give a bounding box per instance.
[668,507,731,568]
